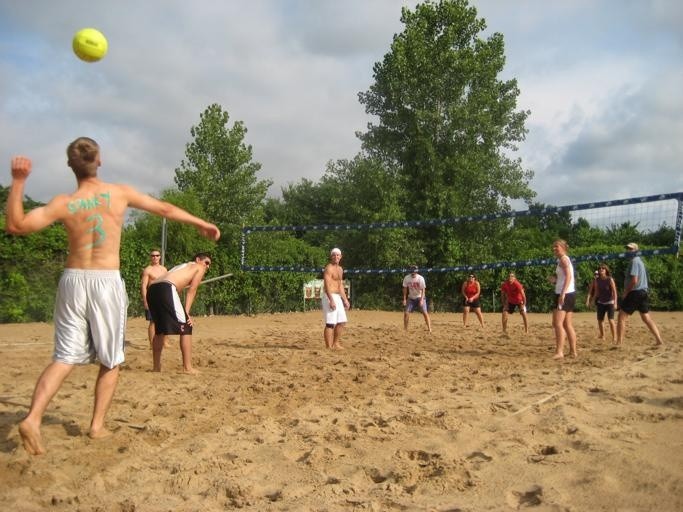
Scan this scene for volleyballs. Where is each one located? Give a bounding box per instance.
[73,28,106,61]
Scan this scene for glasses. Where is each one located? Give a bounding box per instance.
[151,255,159,257]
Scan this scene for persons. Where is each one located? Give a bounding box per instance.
[4,137,220,457]
[611,241,663,350]
[149,253,213,376]
[320,245,351,352]
[584,270,599,306]
[400,263,432,333]
[460,273,485,331]
[591,264,618,345]
[139,248,172,352]
[548,273,568,339]
[549,238,579,359]
[498,270,529,336]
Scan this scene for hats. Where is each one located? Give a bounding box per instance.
[624,242,638,249]
[331,248,341,256]
